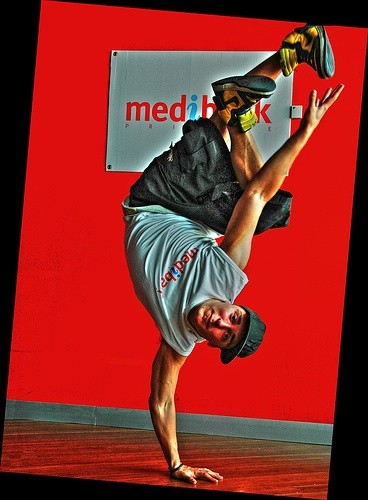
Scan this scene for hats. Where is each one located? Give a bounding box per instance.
[220,305,266,364]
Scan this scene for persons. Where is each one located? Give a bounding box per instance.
[122,24,345,485]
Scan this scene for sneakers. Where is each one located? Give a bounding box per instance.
[278,24,335,79]
[211,75,276,133]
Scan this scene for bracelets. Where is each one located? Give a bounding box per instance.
[168,463,183,473]
